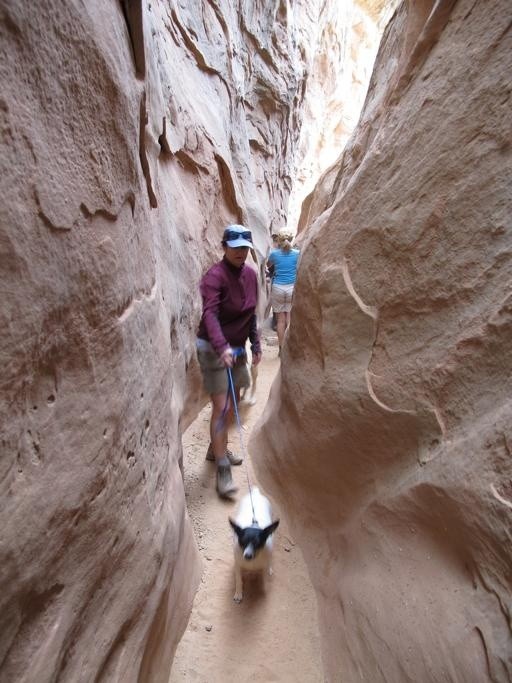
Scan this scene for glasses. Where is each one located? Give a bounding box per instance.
[222,230,251,241]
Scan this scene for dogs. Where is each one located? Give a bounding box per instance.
[228,484,279,604]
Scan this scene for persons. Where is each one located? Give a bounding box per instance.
[193,220,264,500]
[269,226,301,358]
[264,233,280,332]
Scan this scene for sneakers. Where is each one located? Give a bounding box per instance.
[216,463,241,501]
[205,442,242,465]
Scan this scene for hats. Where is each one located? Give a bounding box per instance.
[276,228,293,242]
[222,224,254,250]
